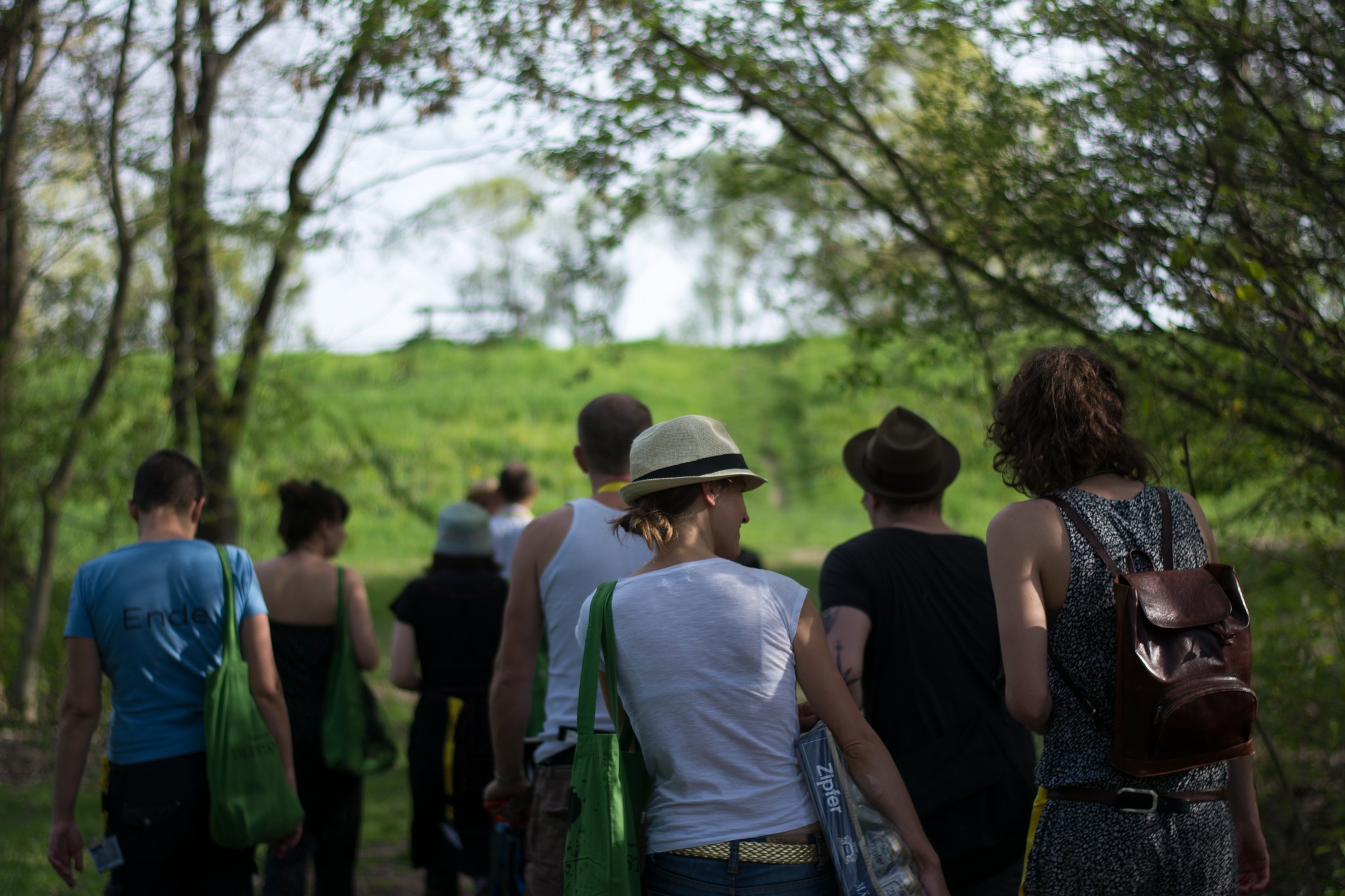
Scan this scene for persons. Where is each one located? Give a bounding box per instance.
[984,342,1273,896]
[573,415,953,896]
[816,406,1038,896]
[467,461,540,580]
[482,394,657,896]
[390,500,512,896]
[46,448,303,896]
[250,480,380,896]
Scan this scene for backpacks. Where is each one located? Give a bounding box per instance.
[1037,485,1258,781]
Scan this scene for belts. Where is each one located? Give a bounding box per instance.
[1046,783,1232,814]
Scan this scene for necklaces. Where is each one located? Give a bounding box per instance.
[597,481,631,493]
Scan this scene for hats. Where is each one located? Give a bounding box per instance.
[432,501,498,558]
[842,405,961,499]
[619,413,769,503]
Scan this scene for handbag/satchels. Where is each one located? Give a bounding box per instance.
[203,647,304,852]
[565,725,635,896]
[319,638,401,775]
